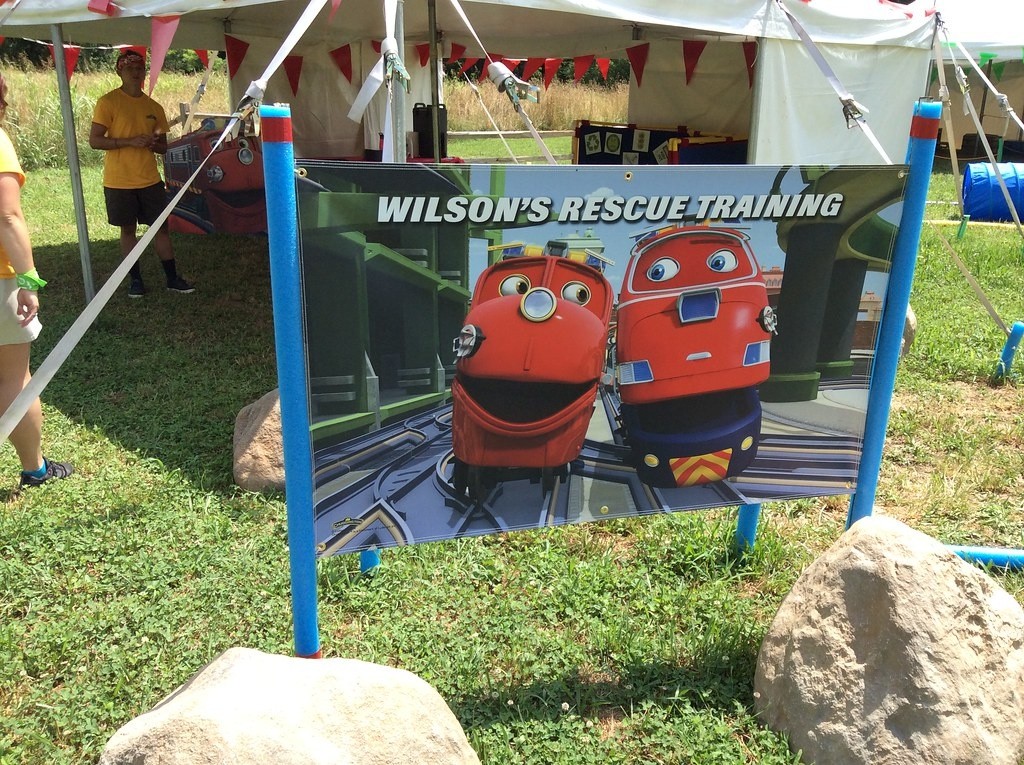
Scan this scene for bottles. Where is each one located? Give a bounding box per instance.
[379,132,384,150]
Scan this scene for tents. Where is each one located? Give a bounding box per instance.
[0,0,1024,324]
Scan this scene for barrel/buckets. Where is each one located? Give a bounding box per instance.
[962,162,1024,223]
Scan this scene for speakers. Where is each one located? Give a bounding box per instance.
[413,103,447,158]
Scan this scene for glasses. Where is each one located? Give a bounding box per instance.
[1,99,8,107]
[121,65,147,73]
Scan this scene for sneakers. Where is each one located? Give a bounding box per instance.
[19,457,72,491]
[166,276,195,293]
[127,276,145,298]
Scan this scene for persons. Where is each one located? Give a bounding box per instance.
[88,52,199,299]
[0,71,72,487]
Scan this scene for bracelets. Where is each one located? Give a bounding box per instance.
[16,267,47,290]
[114,137,120,150]
